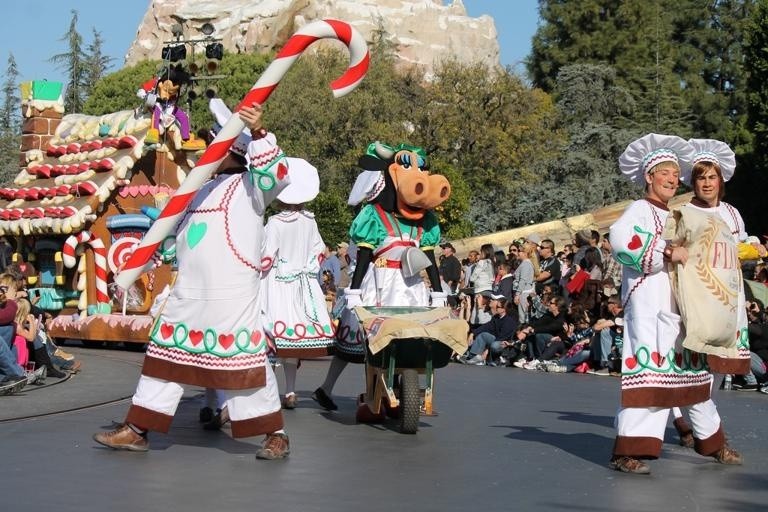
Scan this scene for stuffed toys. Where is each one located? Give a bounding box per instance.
[344,142,453,435]
[137,61,190,155]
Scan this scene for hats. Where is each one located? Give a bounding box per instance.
[679,137,736,189]
[275,157,320,206]
[618,133,696,194]
[337,242,350,249]
[207,97,254,157]
[577,229,592,242]
[439,243,453,250]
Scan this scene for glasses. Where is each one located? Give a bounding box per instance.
[540,244,550,249]
[549,301,555,305]
[508,248,517,252]
[518,248,526,252]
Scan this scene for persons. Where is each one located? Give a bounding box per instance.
[0,233,82,398]
[315,228,768,394]
[197,388,231,429]
[258,197,322,410]
[607,158,743,476]
[91,102,292,462]
[311,181,385,412]
[671,159,768,448]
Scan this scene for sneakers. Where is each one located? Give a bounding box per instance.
[0,376,28,395]
[52,349,74,362]
[204,407,230,428]
[738,384,759,391]
[680,434,695,447]
[453,351,622,376]
[62,360,81,371]
[732,382,747,388]
[281,394,297,409]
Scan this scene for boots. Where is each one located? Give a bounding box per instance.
[34,343,67,378]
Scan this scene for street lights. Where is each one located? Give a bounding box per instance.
[161,14,227,130]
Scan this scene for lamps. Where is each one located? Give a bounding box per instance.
[184,55,219,101]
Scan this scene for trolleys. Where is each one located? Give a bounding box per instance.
[351,304,448,433]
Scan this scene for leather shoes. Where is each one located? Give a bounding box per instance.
[94,422,150,452]
[705,446,743,464]
[610,454,650,474]
[255,434,290,459]
[311,387,337,410]
